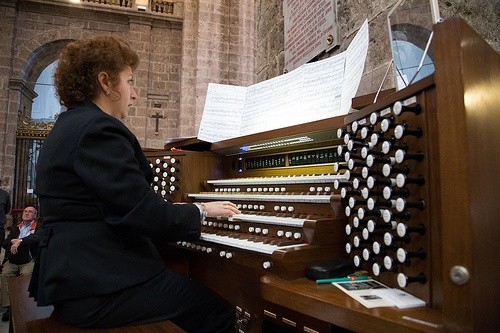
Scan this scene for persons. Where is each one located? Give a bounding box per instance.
[1,206,39,278]
[24,34,242,333]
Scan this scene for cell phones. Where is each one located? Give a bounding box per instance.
[305,260,353,280]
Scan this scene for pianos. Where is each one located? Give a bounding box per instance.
[141,17,500,333]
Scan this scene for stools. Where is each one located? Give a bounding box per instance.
[8,275,186,332]
